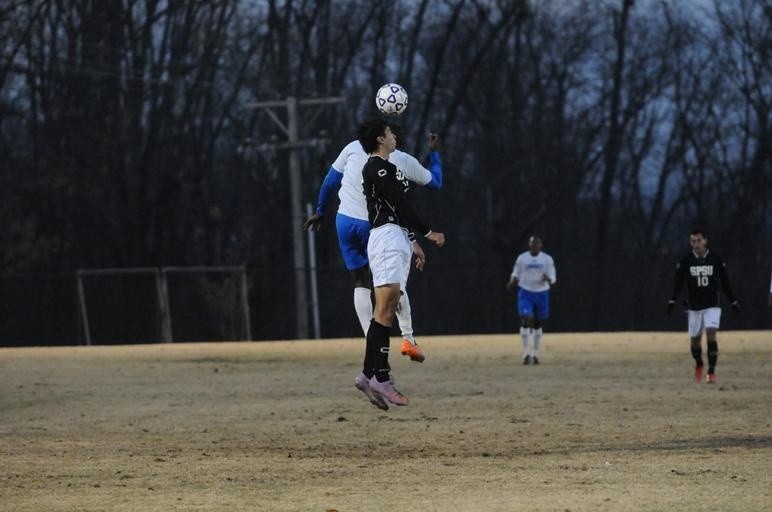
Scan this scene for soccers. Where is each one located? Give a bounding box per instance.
[376,83,410,117]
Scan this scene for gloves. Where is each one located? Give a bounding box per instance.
[666,302,675,313]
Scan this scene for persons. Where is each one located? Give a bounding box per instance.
[304,131,444,362]
[508,236,557,364]
[667,229,740,382]
[353,116,447,409]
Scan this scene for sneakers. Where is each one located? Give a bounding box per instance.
[400,337,425,364]
[694,367,716,385]
[522,355,540,365]
[355,372,409,410]
